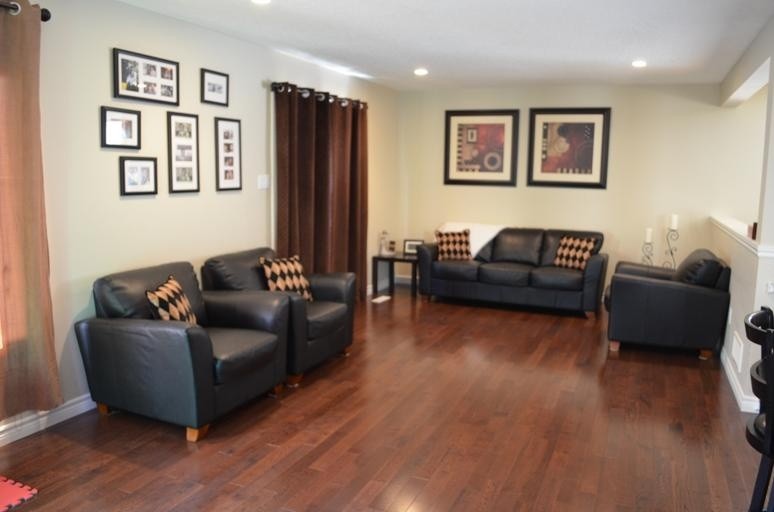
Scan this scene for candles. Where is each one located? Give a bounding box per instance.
[644,227,653,243]
[669,214,677,230]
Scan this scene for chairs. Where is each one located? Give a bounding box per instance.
[201,247,359,392]
[604,246,732,361]
[74,260,291,446]
[739,302,773,511]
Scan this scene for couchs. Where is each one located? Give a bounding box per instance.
[418,221,611,321]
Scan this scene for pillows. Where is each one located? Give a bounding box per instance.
[258,253,314,301]
[434,228,471,262]
[552,234,597,274]
[145,273,198,324]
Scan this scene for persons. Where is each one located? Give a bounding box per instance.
[127,64,172,96]
[177,168,191,181]
[128,167,149,184]
[176,123,190,137]
[177,149,190,161]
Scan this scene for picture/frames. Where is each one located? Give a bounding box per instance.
[527,107,610,189]
[444,109,518,186]
[101,106,142,150]
[113,46,180,107]
[200,67,229,107]
[167,110,200,192]
[214,116,242,191]
[402,239,424,255]
[119,156,157,196]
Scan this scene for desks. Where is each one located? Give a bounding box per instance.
[373,254,422,298]
